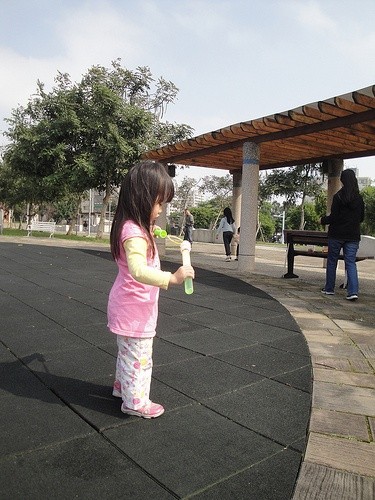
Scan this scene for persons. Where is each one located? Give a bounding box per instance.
[233,227,241,262]
[183,209,194,247]
[108,159,195,420]
[318,170,364,301]
[83,221,88,232]
[216,207,236,262]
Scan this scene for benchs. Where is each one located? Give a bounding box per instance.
[27,221,56,238]
[282,229,367,288]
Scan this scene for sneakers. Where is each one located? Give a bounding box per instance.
[345,294,358,299]
[112,383,122,397]
[121,401,164,419]
[321,288,334,294]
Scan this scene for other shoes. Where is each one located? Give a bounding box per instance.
[225,257,238,261]
